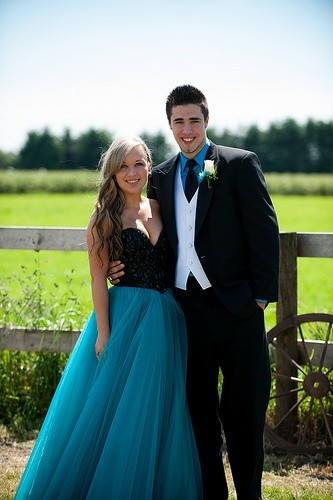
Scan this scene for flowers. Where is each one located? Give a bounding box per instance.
[196,160,218,188]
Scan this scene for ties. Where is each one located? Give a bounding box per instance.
[185,161,202,203]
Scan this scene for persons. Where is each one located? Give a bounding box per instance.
[13,136,204,500]
[147,85,279,500]
